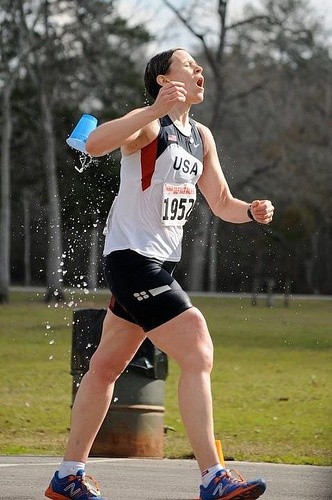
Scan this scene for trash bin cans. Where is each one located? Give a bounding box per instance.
[70,307,168,461]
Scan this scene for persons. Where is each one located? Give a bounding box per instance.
[44,47,274,500]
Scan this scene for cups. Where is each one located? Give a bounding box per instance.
[66,114,98,153]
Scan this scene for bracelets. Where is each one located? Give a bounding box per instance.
[247,203,260,222]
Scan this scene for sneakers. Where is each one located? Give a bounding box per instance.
[45,469,106,500]
[199,468,265,500]
